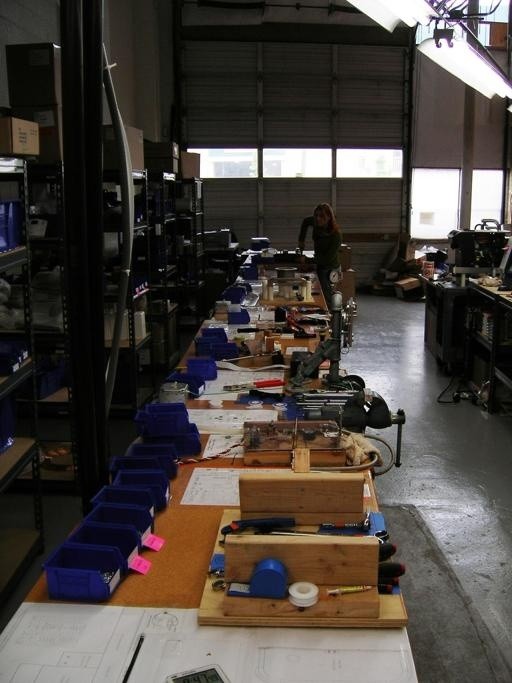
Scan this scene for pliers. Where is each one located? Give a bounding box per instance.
[223,379,286,391]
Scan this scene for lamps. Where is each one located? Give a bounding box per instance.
[346,0,512,101]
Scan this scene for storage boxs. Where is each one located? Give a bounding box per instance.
[336,243,357,305]
[0,41,63,155]
[103,124,200,180]
[380,232,417,271]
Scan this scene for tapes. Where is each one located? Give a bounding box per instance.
[288,582,319,607]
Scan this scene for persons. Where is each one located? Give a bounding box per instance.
[298,202,343,307]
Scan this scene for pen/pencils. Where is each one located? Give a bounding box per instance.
[328,585,372,594]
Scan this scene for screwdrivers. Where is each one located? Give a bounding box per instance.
[237,328,265,333]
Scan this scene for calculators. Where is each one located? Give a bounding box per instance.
[167,665,230,683]
